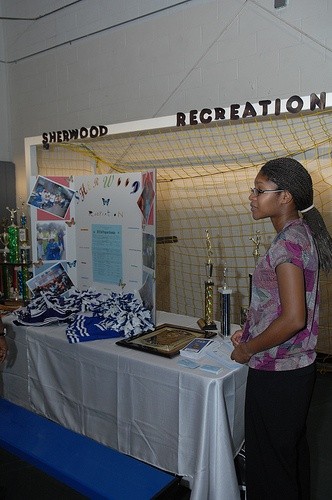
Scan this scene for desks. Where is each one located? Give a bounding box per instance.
[0,297,252,483]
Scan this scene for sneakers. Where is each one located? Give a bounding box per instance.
[236,471,247,491]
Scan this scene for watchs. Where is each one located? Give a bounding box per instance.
[0,327,7,336]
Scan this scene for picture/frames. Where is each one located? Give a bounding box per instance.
[116,322,210,359]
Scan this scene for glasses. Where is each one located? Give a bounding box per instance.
[250,187,284,195]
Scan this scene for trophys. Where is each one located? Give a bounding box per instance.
[0,199,33,307]
[217,261,234,341]
[237,228,263,331]
[197,228,218,331]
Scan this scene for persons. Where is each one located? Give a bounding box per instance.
[32,272,71,298]
[0,316,8,366]
[37,225,65,260]
[30,187,68,210]
[228,156,332,500]
[145,242,153,268]
